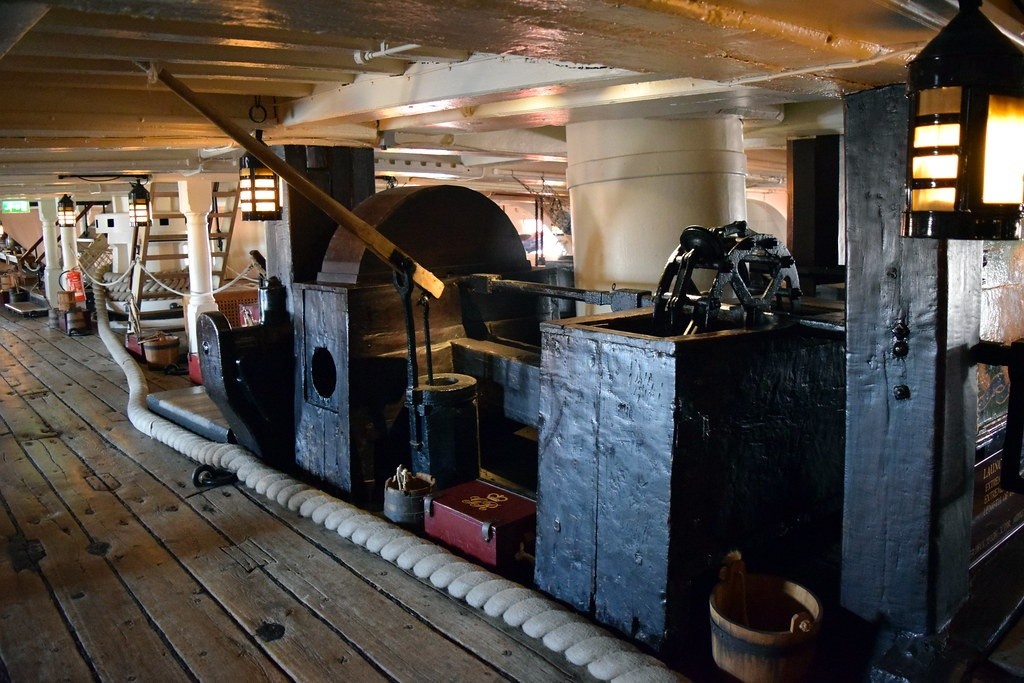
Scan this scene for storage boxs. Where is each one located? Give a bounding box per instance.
[187,353,203,386]
[428,475,537,578]
[129,332,172,363]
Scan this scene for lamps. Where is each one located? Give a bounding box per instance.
[128,179,153,228]
[58,193,76,227]
[236,128,283,224]
[898,0,1024,242]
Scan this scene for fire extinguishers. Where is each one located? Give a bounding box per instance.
[58,265,86,303]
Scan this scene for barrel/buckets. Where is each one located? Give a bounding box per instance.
[67,311,86,336]
[9,288,29,303]
[709,575,823,683]
[48,309,59,328]
[57,290,76,310]
[143,332,179,371]
[384,472,436,523]
